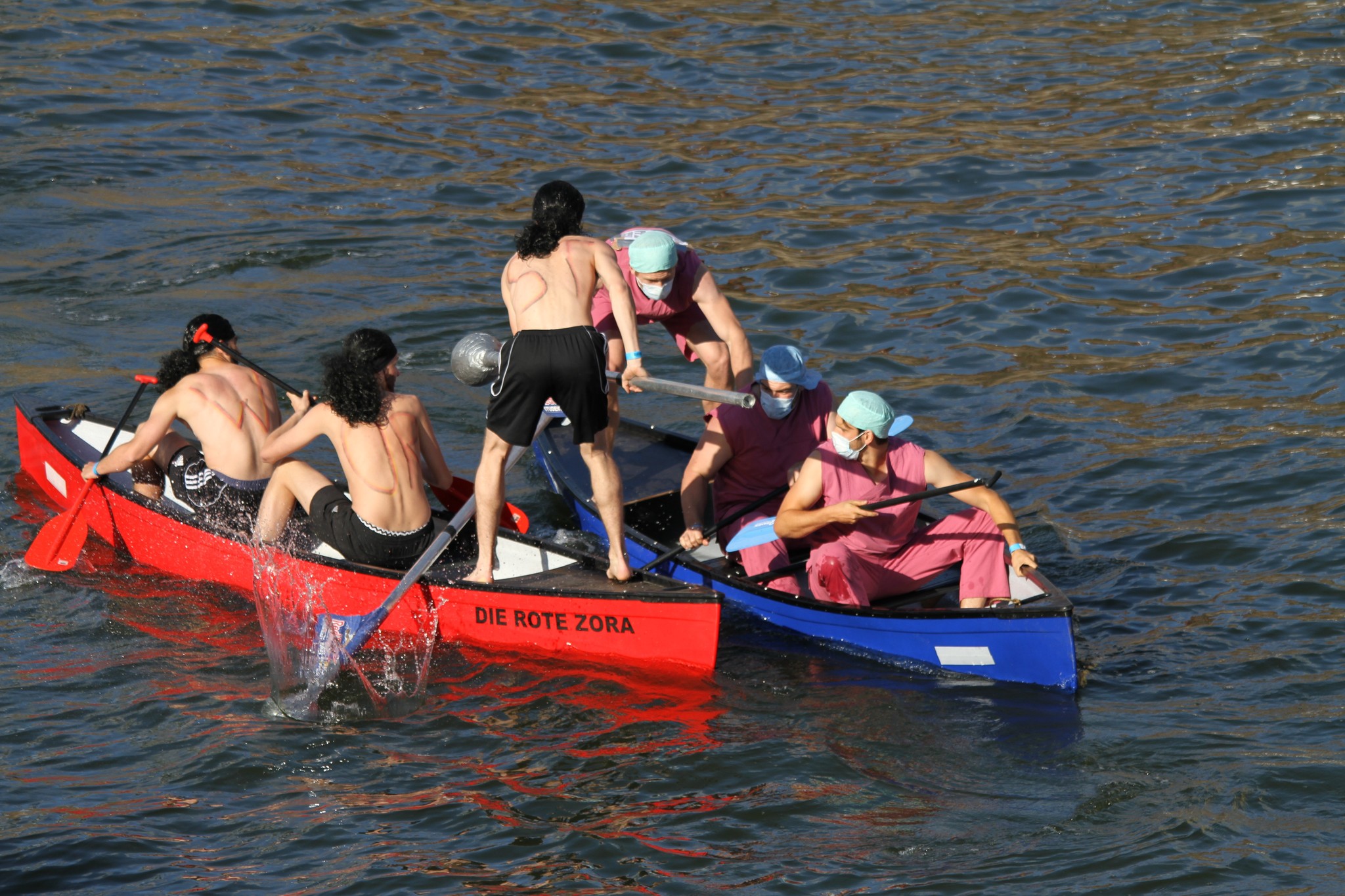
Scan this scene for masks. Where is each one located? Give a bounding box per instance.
[831,431,867,460]
[760,383,799,420]
[636,277,673,300]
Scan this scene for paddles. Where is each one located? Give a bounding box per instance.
[25,375,157,572]
[637,485,789,572]
[726,471,1001,554]
[194,321,529,533]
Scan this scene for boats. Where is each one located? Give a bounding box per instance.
[8,389,725,674]
[532,400,1078,692]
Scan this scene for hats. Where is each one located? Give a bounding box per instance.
[628,230,678,274]
[756,345,822,390]
[837,390,895,439]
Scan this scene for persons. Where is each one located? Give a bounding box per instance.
[81,312,286,536]
[772,390,1037,609]
[460,181,650,584]
[252,327,453,571]
[679,344,836,599]
[590,228,754,443]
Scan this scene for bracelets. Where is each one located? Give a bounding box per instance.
[1008,543,1027,555]
[625,351,643,361]
[691,524,703,529]
[93,462,101,476]
